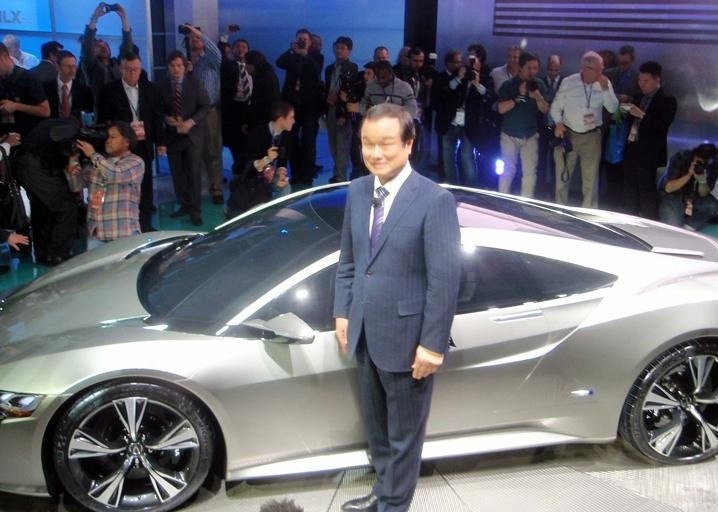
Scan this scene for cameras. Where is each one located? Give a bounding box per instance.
[527,81,537,92]
[545,121,562,151]
[274,135,289,169]
[339,71,364,101]
[297,39,305,48]
[178,23,200,34]
[695,160,705,175]
[456,66,476,79]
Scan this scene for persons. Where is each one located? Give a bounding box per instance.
[0,0,718,265]
[332,103,461,512]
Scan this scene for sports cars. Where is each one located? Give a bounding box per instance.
[1,175,717,506]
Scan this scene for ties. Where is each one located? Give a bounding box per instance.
[241,66,250,106]
[629,94,651,141]
[61,84,71,120]
[370,187,389,260]
[173,81,182,121]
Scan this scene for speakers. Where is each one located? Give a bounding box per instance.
[405,0,439,66]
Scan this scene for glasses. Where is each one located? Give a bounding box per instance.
[583,64,605,75]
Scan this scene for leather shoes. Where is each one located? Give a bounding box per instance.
[212,195,224,204]
[191,213,203,226]
[172,206,188,217]
[341,493,378,512]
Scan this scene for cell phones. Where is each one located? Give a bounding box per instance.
[230,24,239,32]
[619,106,632,112]
[105,5,118,11]
[469,55,475,68]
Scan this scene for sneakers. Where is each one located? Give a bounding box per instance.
[328,178,346,183]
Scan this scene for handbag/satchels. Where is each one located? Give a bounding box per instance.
[226,176,271,210]
[1,180,30,233]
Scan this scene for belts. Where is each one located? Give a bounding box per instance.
[563,124,602,134]
